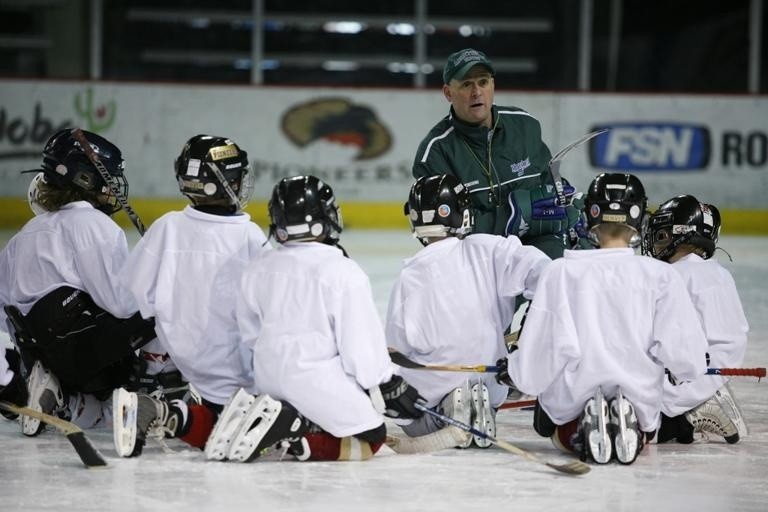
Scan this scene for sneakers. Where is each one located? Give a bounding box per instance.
[26,369,68,438]
[442,387,474,448]
[684,394,740,445]
[240,398,311,462]
[471,384,497,448]
[609,397,644,465]
[125,393,171,457]
[572,396,612,465]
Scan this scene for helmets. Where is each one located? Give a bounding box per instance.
[173,133,257,211]
[580,172,648,247]
[403,172,476,241]
[261,175,350,259]
[643,195,722,260]
[25,171,50,215]
[19,127,130,215]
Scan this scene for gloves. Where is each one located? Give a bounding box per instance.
[366,374,430,420]
[502,176,575,239]
[495,356,517,389]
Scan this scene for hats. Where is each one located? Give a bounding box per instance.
[442,47,494,85]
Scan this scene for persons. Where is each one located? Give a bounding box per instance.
[0,128,128,438]
[384,173,546,450]
[506,173,708,464]
[112,134,272,458]
[412,49,588,260]
[204,175,412,465]
[646,195,749,445]
